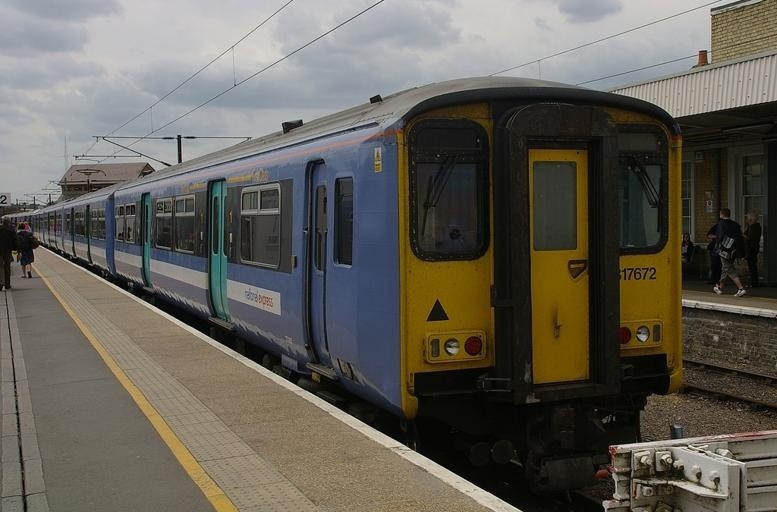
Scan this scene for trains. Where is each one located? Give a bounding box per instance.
[0,75,692,497]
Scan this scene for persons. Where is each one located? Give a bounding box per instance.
[706,223,724,286]
[743,212,763,286]
[681,230,695,265]
[16,222,40,279]
[712,207,749,297]
[0,215,16,291]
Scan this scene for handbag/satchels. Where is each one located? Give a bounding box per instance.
[30,238,39,249]
[716,234,736,260]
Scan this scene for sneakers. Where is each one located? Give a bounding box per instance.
[713,286,722,295]
[28,271,32,278]
[21,275,26,278]
[5,285,12,289]
[734,289,745,297]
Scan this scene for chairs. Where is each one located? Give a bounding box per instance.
[682,245,701,273]
